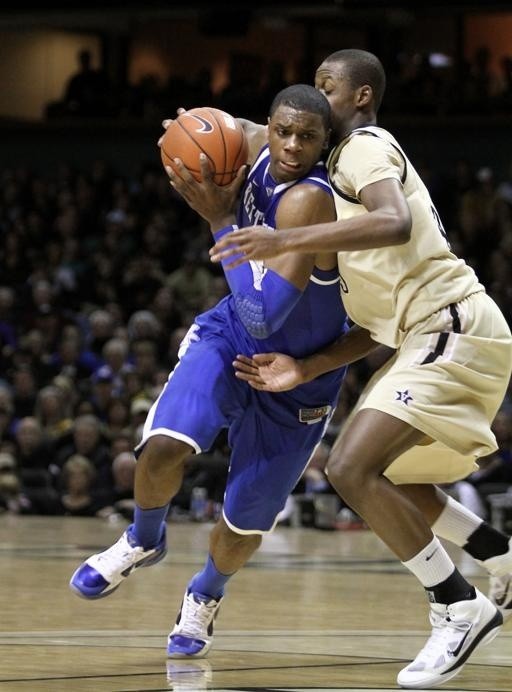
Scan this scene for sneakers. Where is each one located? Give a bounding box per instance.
[164,568,225,661]
[487,552,512,610]
[395,584,506,690]
[68,520,169,604]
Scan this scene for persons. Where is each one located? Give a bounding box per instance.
[207,47,512,688]
[1,1,512,541]
[65,81,350,662]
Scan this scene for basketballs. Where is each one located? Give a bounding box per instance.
[162,107,248,186]
